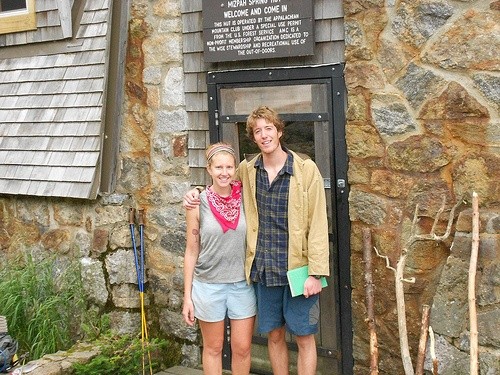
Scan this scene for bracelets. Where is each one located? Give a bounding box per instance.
[196,188,201,195]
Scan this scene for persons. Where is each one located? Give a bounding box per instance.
[182,141,257,375]
[183,105,330,375]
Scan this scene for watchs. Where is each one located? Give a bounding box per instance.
[311,275,321,279]
